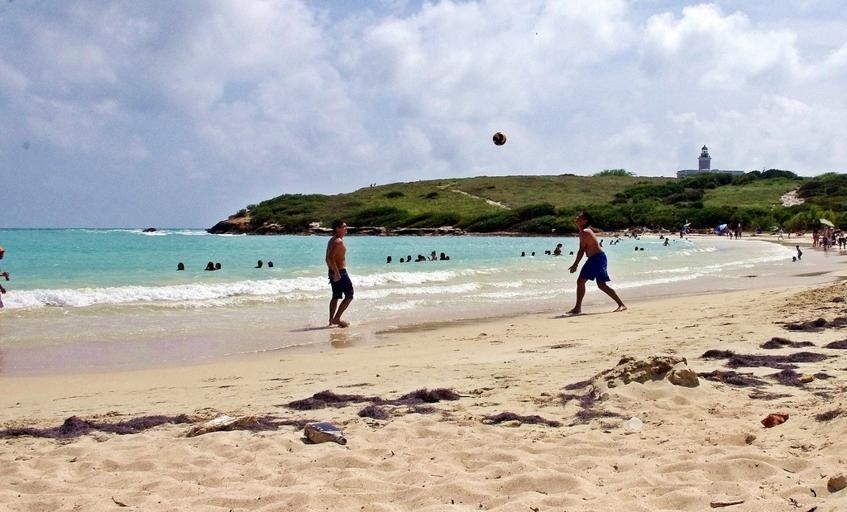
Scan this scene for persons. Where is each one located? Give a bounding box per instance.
[176,262,185,271]
[521,243,573,256]
[215,262,221,269]
[254,260,263,268]
[325,219,354,328]
[205,262,217,271]
[565,211,627,314]
[599,223,847,262]
[268,261,273,267]
[0,247,10,309]
[386,250,450,263]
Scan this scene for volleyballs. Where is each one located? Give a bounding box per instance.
[493,132,506,145]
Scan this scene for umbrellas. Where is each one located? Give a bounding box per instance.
[819,218,835,227]
